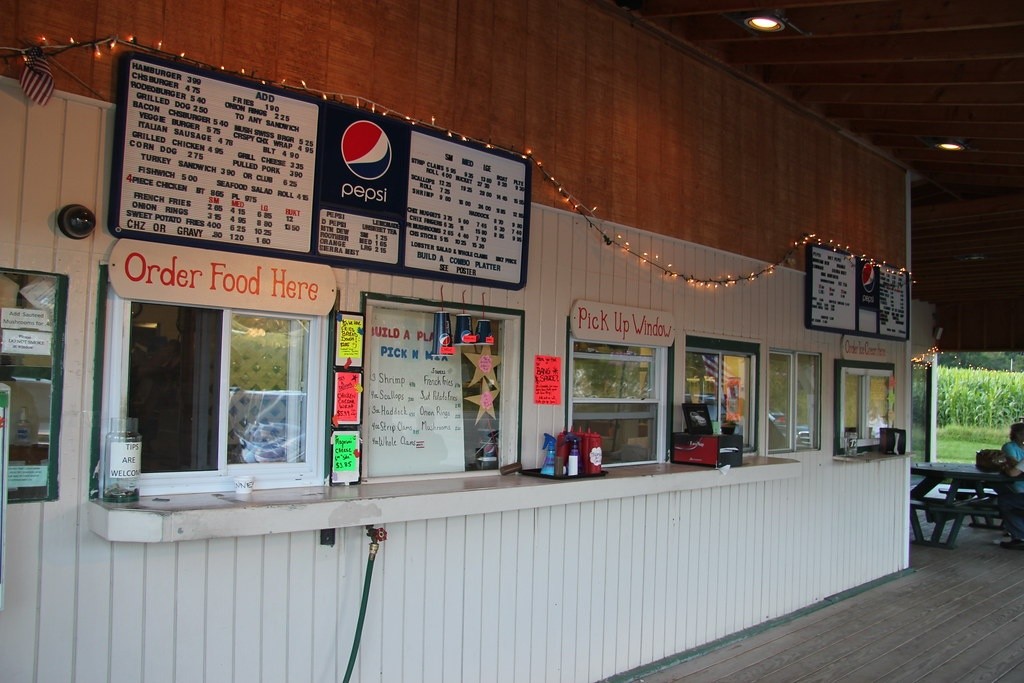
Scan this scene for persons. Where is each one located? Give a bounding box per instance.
[997,422,1024,549]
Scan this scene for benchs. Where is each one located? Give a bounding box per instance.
[910,489,1003,517]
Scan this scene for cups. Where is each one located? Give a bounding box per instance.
[485,443,495,456]
[473,320,495,346]
[454,314,474,347]
[431,312,455,357]
[232,475,254,494]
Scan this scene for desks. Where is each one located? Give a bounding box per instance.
[910,462,1024,549]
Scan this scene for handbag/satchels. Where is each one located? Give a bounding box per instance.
[976,450,1008,472]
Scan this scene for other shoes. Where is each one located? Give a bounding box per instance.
[1003,528,1012,536]
[1000,538,1024,550]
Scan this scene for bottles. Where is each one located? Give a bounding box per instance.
[844,427,858,456]
[102,417,142,503]
[556,425,603,473]
